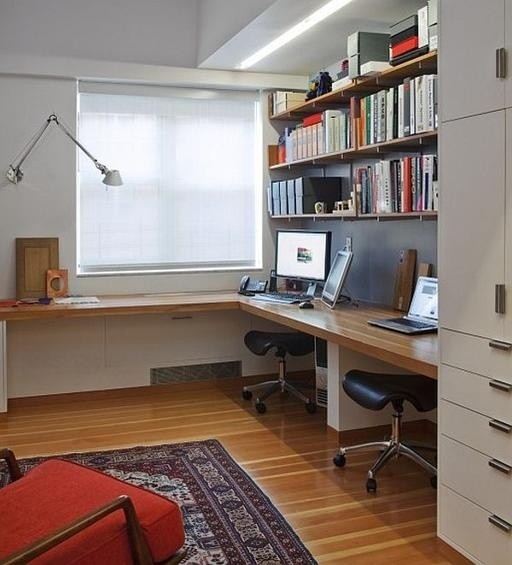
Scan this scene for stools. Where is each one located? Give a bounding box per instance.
[331,369,438,495]
[244,331,318,415]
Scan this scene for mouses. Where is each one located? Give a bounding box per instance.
[298,302,314,308]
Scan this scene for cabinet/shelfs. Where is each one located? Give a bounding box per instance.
[266,51,438,221]
[436,107,512,345]
[436,326,512,565]
[437,0,512,124]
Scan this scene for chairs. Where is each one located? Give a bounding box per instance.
[0,446,185,565]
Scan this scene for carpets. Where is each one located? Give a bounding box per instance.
[1,440,318,565]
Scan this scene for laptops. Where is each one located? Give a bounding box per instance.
[367,276,438,335]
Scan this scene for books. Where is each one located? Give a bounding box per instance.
[270,1,437,216]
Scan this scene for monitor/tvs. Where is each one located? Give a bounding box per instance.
[320,250,359,310]
[275,228,332,299]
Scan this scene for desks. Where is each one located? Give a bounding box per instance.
[0,289,442,431]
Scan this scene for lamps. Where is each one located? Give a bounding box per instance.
[6,114,124,186]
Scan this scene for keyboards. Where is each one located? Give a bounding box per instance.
[252,292,314,304]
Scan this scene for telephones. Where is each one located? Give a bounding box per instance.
[238,275,268,293]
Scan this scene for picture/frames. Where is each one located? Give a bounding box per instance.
[15,237,59,302]
